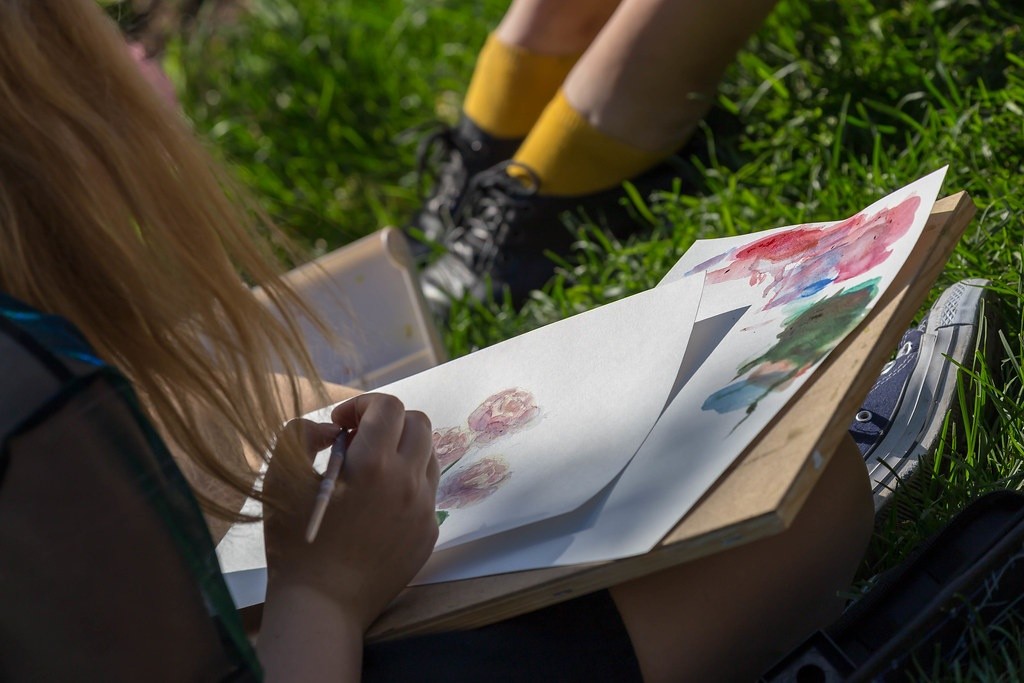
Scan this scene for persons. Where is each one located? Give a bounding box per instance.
[394,0,777,324]
[0,0,1000,683]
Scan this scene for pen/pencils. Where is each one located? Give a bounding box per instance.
[302,429,352,544]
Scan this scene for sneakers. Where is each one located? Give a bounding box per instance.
[851,279,991,530]
[403,103,722,319]
